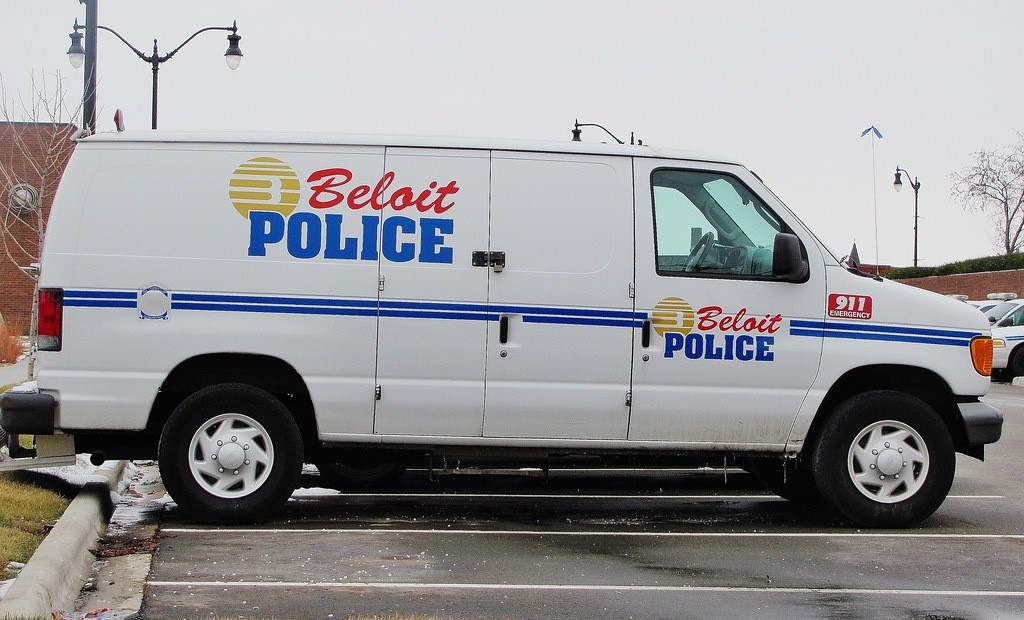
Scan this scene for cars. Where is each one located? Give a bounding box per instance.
[946,291,1024,380]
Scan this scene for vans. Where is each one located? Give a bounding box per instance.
[0,131,1006,525]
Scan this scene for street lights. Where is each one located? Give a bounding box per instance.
[68,17,243,132]
[893,163,921,268]
